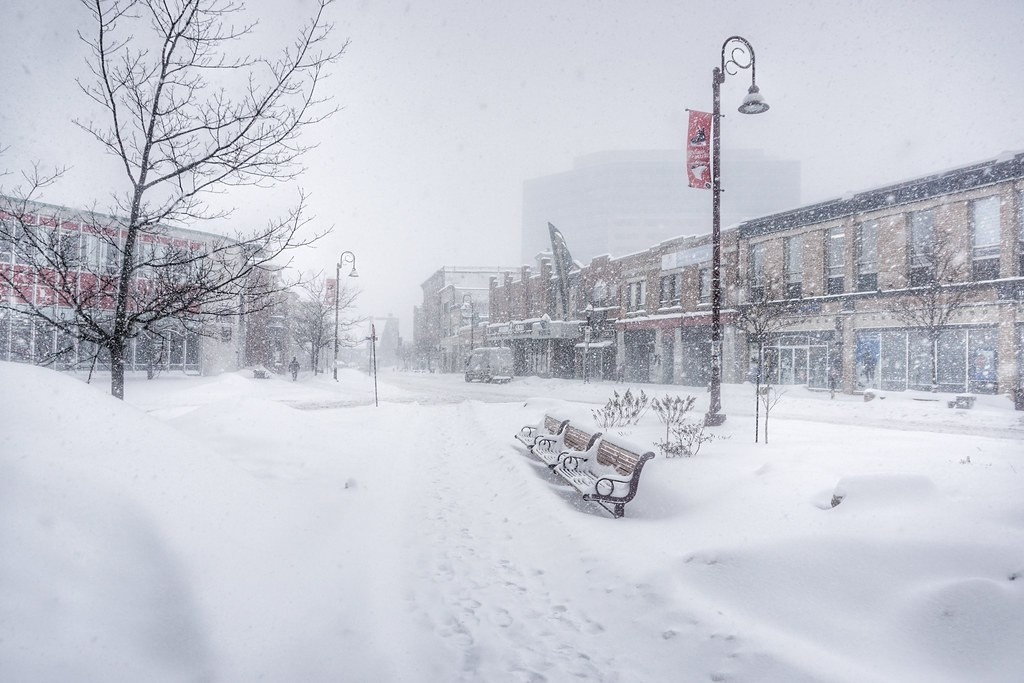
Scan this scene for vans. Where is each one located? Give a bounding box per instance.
[465,347,515,383]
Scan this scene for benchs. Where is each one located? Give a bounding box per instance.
[514,416,655,521]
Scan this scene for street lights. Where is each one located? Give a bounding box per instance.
[584,304,594,383]
[334,251,359,379]
[460,294,473,350]
[705,36,770,425]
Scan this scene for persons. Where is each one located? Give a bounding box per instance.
[289,357,300,382]
[828,370,837,400]
